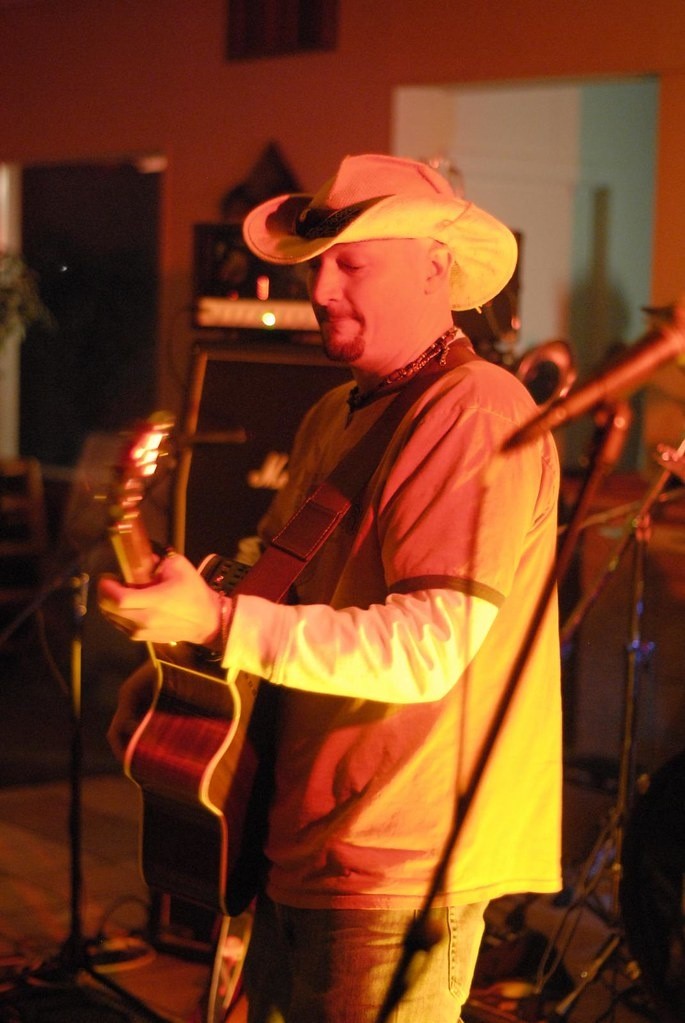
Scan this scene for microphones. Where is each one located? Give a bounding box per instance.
[502,302,685,461]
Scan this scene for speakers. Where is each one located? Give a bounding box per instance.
[137,352,352,904]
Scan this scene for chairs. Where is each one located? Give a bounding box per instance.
[0,457,66,697]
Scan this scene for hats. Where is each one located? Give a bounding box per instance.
[243,154,518,311]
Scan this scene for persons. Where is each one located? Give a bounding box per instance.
[97,153,567,1023]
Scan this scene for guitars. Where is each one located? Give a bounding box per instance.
[70,402,302,918]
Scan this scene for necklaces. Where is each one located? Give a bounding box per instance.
[346,326,458,430]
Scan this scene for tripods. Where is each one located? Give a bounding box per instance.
[520,453,685,1023]
[0,565,173,1023]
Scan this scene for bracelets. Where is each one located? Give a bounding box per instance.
[213,594,232,652]
